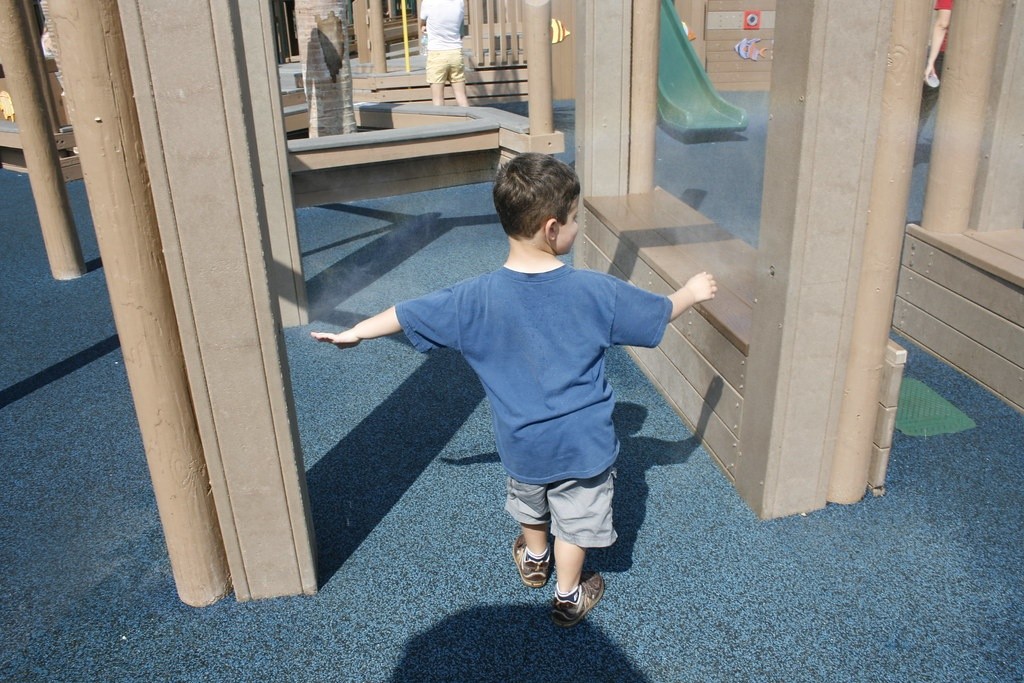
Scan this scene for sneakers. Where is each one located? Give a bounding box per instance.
[551,570,605,627]
[513,534,550,588]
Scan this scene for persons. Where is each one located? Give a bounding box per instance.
[310,151,719,630]
[924,0,955,86]
[419,0,469,108]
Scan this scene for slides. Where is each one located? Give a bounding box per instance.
[658,0,749,134]
